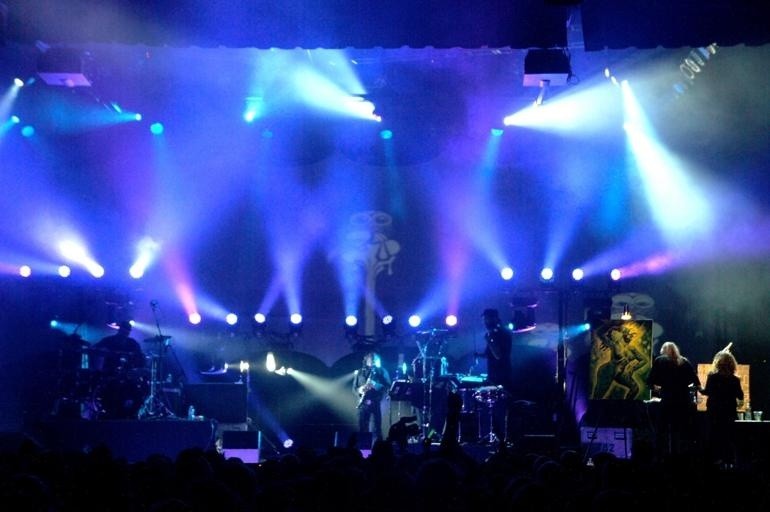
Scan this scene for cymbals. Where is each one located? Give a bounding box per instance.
[419,329,450,333]
[144,336,171,342]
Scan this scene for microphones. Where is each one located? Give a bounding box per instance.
[149,299,158,306]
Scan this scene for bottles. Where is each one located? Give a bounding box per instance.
[188,405,195,420]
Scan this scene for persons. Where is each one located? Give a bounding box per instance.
[473,308,513,437]
[646,341,744,420]
[1,439,770,511]
[352,353,391,448]
[92,323,144,374]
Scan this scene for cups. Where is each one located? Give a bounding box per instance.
[738,409,763,420]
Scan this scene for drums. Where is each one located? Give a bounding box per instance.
[71,345,104,371]
[95,372,145,419]
[453,389,476,415]
[473,386,507,407]
[412,356,448,378]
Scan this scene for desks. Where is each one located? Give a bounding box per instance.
[105,412,219,459]
[714,417,768,459]
